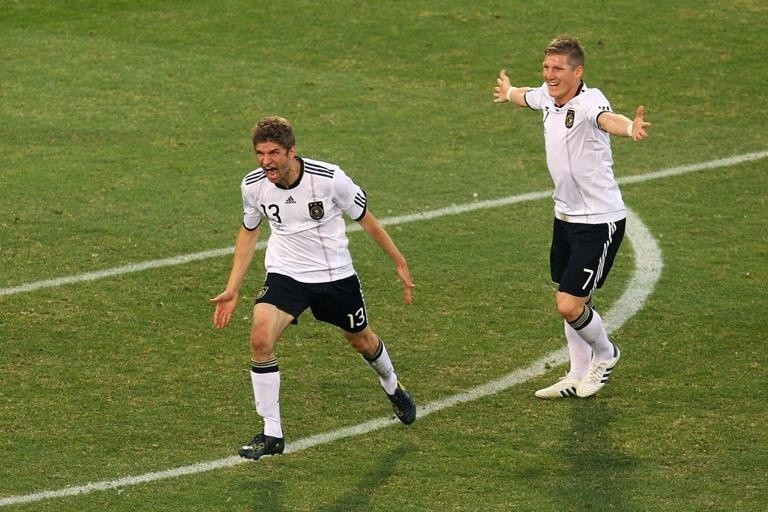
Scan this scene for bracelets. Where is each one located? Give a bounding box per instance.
[506,86,515,101]
[627,120,634,138]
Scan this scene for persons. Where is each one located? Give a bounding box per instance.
[492,35,654,398]
[210,115,417,459]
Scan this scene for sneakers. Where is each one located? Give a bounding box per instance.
[534,376,580,399]
[576,346,621,397]
[239,434,284,460]
[382,382,416,425]
